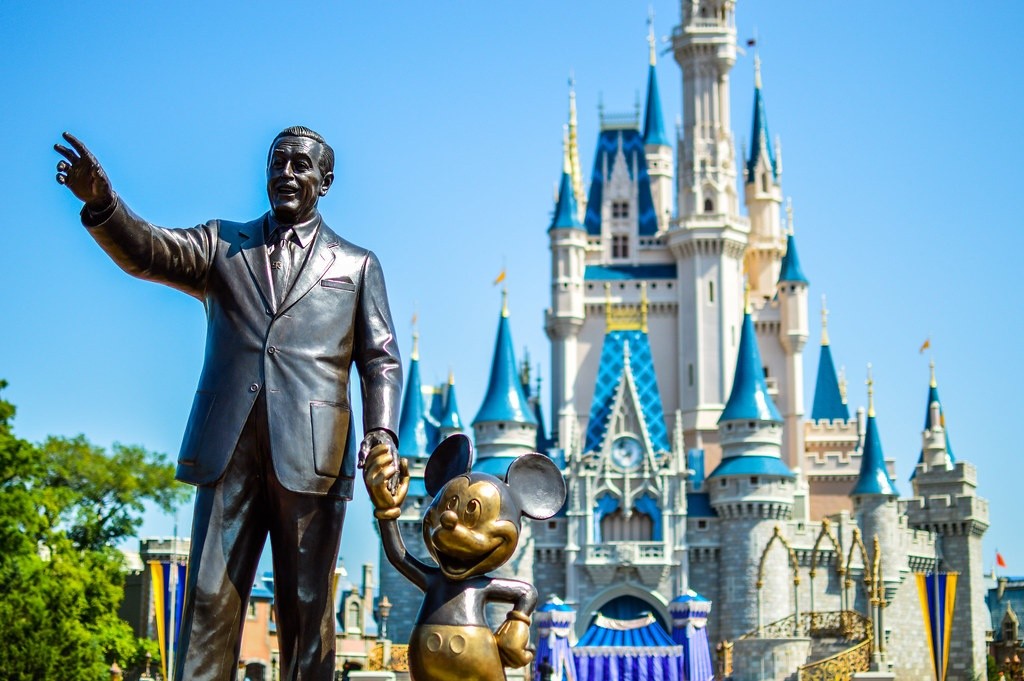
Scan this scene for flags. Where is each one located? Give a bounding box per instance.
[996,548,1006,569]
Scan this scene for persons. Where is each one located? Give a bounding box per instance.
[53,125,403,679]
